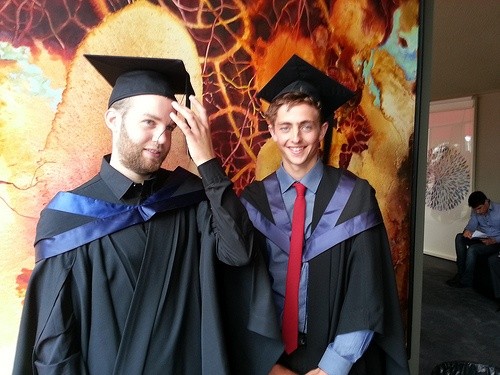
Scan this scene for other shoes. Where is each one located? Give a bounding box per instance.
[448,276,461,284]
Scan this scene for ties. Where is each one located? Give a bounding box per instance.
[282,182,307,354]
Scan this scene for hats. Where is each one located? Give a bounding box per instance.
[256,53,355,166]
[84,54,196,159]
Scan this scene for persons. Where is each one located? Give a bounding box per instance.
[239,54,410,375]
[444,191,500,289]
[12,54,261,375]
